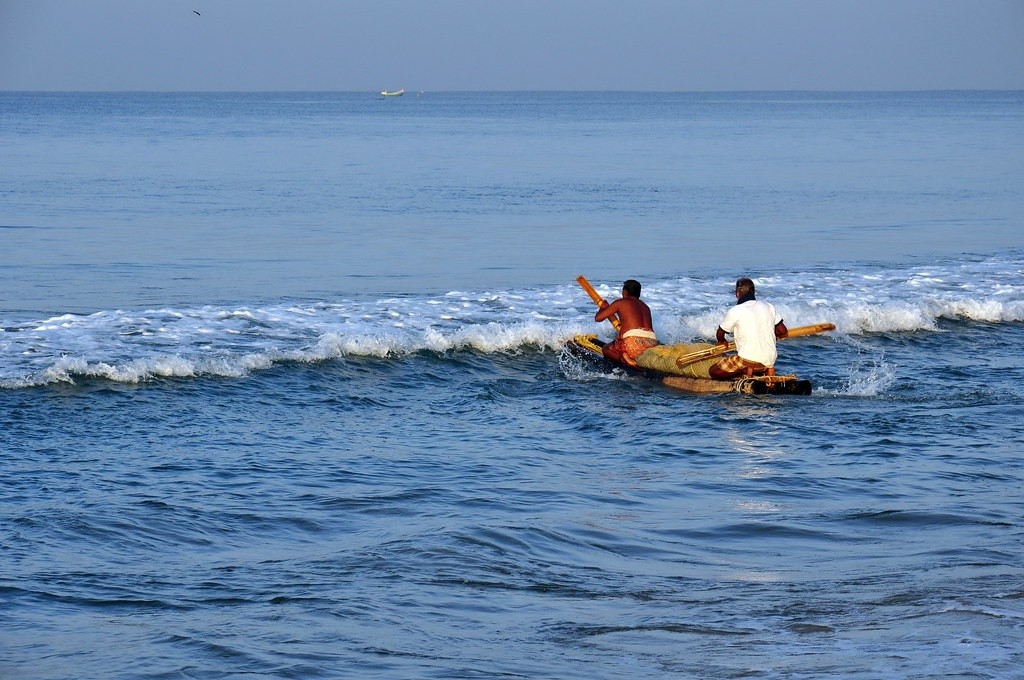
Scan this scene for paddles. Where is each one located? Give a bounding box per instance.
[576,273,622,334]
[675,321,837,367]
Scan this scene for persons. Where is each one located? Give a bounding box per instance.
[594,279,657,367]
[708,277,791,388]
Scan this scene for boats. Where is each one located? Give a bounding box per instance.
[381,89,404,96]
[567,275,812,396]
